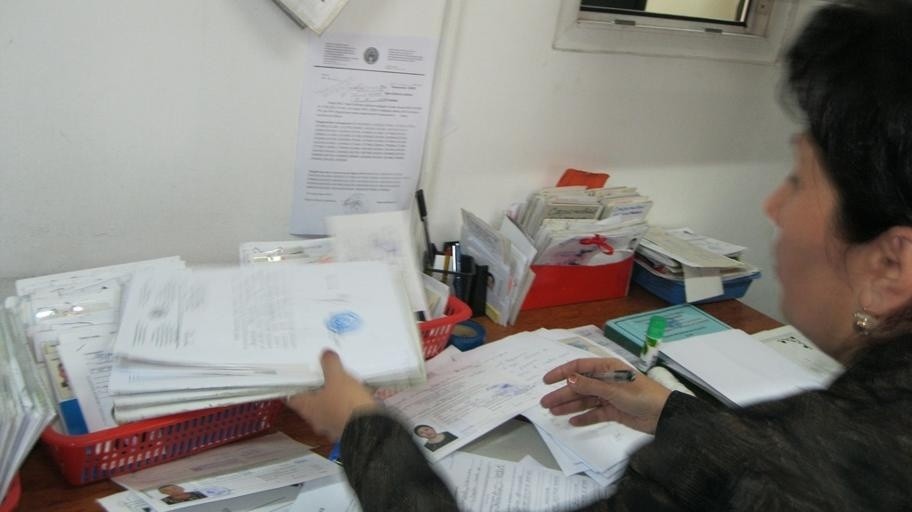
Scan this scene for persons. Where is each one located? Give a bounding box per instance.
[287,1,911,510]
[486,272,497,306]
[156,482,208,506]
[412,424,459,451]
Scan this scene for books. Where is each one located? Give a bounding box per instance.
[10,212,448,467]
[604,301,733,374]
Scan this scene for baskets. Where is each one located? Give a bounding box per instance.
[39,298,470,489]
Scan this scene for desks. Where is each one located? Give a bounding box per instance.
[15,299,847,512]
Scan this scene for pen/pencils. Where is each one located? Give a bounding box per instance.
[581,370,637,381]
[415,189,461,301]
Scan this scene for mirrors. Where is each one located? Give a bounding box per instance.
[553,0,798,66]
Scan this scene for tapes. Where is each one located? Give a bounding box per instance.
[445,319,486,353]
